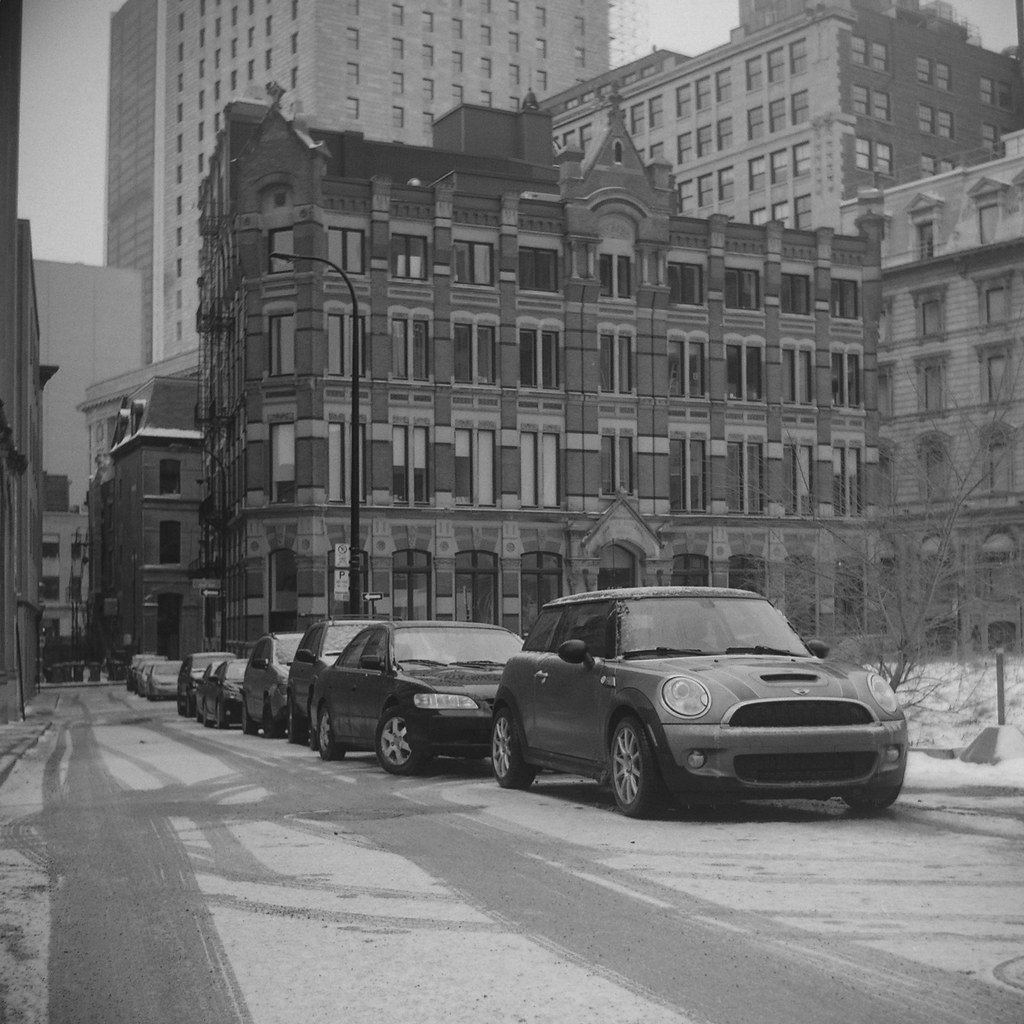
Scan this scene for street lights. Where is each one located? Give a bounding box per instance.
[269,252,363,615]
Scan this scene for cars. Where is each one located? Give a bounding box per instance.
[125,654,183,701]
[194,659,250,729]
[286,619,388,744]
[177,652,238,717]
[312,622,528,775]
[827,635,901,665]
[488,583,909,816]
[242,631,308,738]
